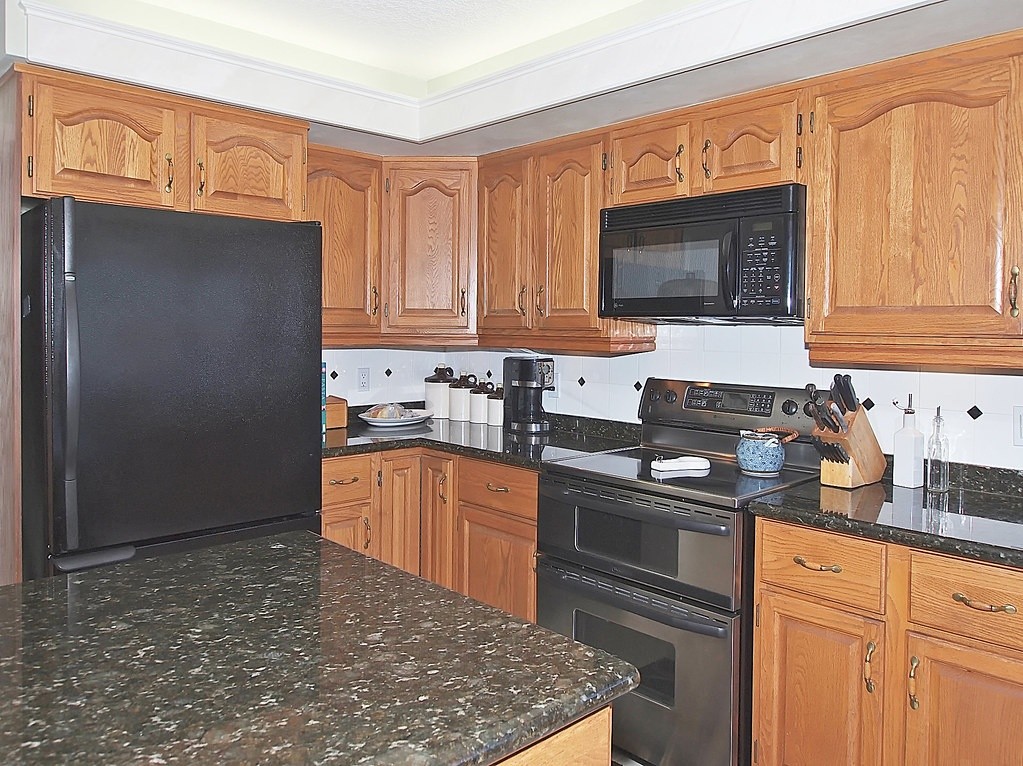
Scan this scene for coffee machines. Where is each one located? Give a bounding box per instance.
[503,356,555,445]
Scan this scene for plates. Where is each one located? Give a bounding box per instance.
[358,410,434,426]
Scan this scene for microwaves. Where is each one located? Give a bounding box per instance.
[599,183,806,327]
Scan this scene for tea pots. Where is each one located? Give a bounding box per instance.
[736,426,799,477]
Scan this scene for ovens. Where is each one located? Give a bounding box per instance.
[536,471,754,766]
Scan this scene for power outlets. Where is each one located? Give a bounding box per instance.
[357,368,370,391]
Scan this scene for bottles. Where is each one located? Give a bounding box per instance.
[449,372,479,421]
[470,379,496,424]
[927,405,949,493]
[893,394,924,488]
[488,383,504,426]
[425,363,458,418]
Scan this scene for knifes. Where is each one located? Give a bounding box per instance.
[808,375,861,464]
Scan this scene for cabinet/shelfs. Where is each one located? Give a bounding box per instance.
[751,515,1023,766]
[320,448,540,627]
[0,64,1023,376]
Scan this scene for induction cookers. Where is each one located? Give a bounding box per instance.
[539,377,831,511]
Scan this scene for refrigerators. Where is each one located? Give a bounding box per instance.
[21,196,322,581]
